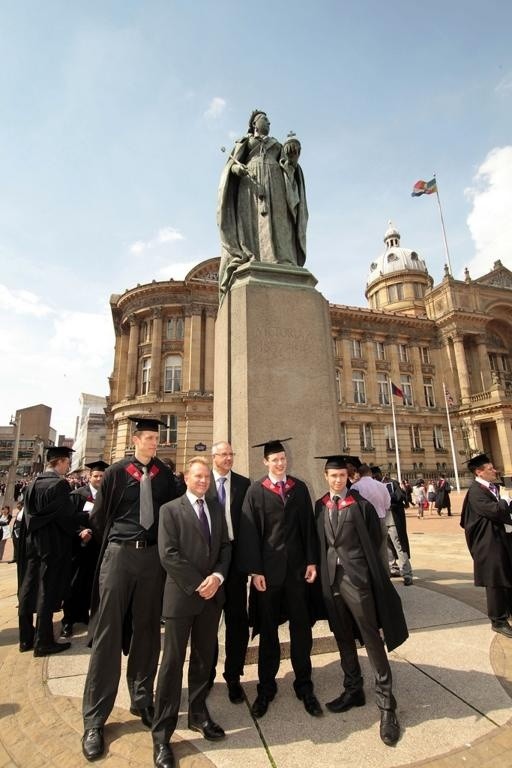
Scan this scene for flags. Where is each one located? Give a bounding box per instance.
[392,382,404,399]
[411,178,438,197]
[444,382,461,407]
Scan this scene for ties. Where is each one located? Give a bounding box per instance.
[330,497,339,536]
[197,499,210,543]
[277,482,287,501]
[139,467,155,530]
[217,478,226,508]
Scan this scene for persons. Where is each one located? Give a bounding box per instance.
[82,415,182,762]
[344,459,453,586]
[459,452,512,637]
[238,438,323,717]
[1,462,111,646]
[313,452,408,746]
[202,440,253,704]
[17,446,78,656]
[218,109,309,303]
[151,456,233,768]
[486,467,501,504]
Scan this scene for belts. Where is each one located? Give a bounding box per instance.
[127,540,153,548]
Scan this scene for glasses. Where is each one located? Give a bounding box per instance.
[216,453,234,457]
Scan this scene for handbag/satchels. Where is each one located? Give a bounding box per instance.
[423,500,428,508]
[428,492,436,502]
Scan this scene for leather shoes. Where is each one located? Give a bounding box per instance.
[404,578,412,585]
[83,727,104,758]
[492,625,512,637]
[187,719,227,740]
[153,742,175,767]
[251,695,275,717]
[325,692,366,714]
[379,710,398,746]
[226,683,245,704]
[129,706,154,729]
[19,624,72,657]
[299,693,324,716]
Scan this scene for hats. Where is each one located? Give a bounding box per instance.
[314,456,355,469]
[128,417,169,432]
[462,452,491,472]
[370,464,383,473]
[85,461,111,470]
[44,446,76,461]
[252,438,292,457]
